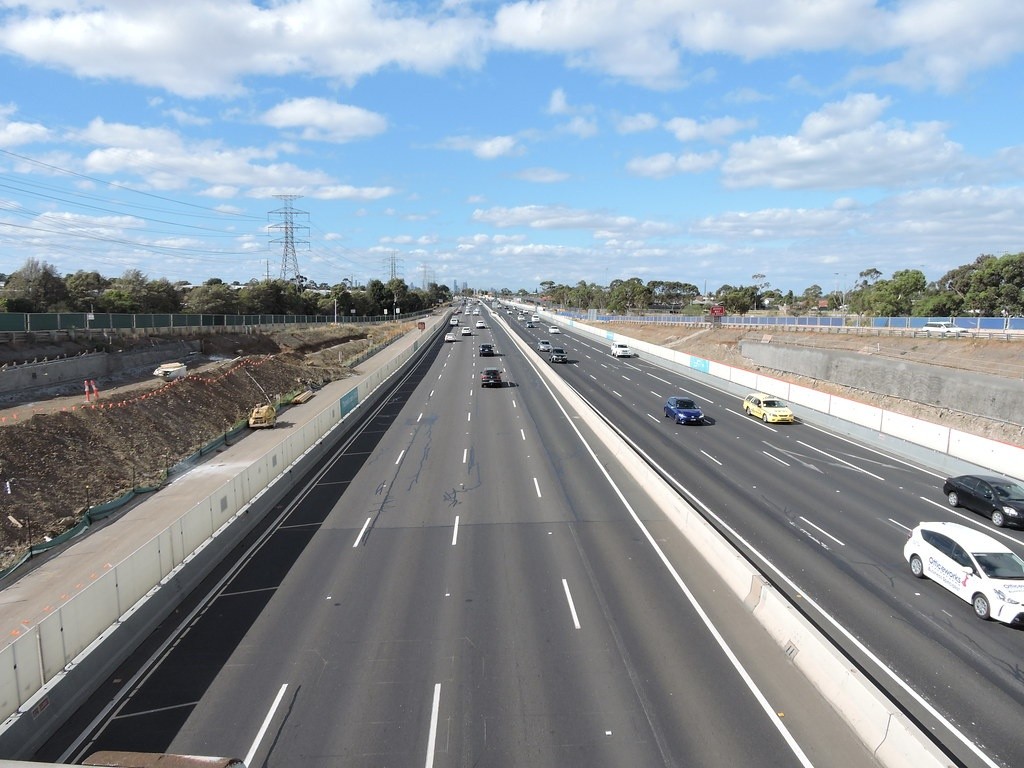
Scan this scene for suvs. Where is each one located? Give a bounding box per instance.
[611,342,630,358]
[922,321,968,338]
[480,367,502,388]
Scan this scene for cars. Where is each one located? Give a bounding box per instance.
[903,520,1024,630]
[549,325,560,334]
[445,333,456,343]
[449,318,459,326]
[743,393,794,424]
[454,299,540,328]
[943,474,1024,531]
[550,347,568,364]
[478,344,494,357]
[460,328,471,336]
[663,396,705,426]
[476,321,485,329]
[452,315,459,322]
[538,340,553,352]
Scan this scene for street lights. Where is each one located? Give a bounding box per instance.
[333,298,337,323]
